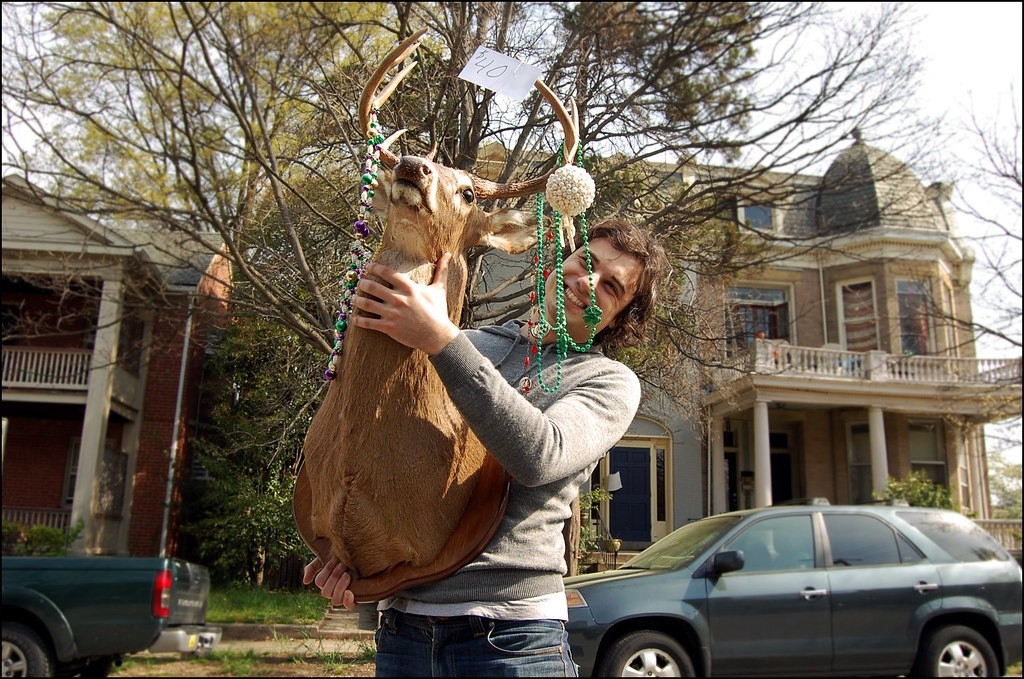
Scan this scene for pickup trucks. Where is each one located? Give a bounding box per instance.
[0,555,222,678]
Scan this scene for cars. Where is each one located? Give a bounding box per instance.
[563,504,1023,678]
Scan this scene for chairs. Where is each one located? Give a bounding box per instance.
[773,551,813,569]
[819,343,848,375]
[750,548,777,569]
[770,339,790,358]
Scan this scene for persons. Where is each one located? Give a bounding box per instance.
[304,217,669,679]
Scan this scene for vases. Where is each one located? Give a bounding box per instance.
[604,539,622,553]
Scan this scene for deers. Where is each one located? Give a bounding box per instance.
[302,25,581,580]
